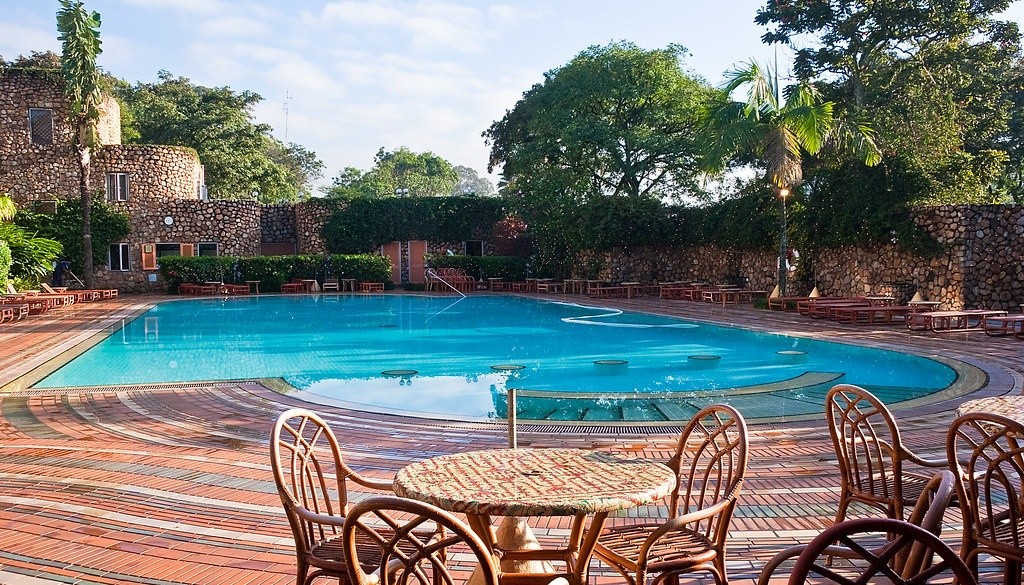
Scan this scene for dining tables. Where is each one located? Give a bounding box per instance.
[956,395,1024,506]
[394,443,679,583]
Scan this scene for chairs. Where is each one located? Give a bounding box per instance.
[786,517,981,585]
[825,384,979,564]
[269,408,445,584]
[944,408,1024,585]
[488,277,503,291]
[341,492,498,585]
[582,402,750,584]
[424,266,477,293]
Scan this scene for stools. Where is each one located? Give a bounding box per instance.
[245,280,261,294]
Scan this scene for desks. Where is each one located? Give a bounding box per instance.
[907,300,943,321]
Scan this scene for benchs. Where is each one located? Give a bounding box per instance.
[0,282,119,324]
[907,309,1008,335]
[768,295,913,325]
[513,277,766,305]
[984,314,1024,339]
[179,280,251,295]
[281,279,385,295]
